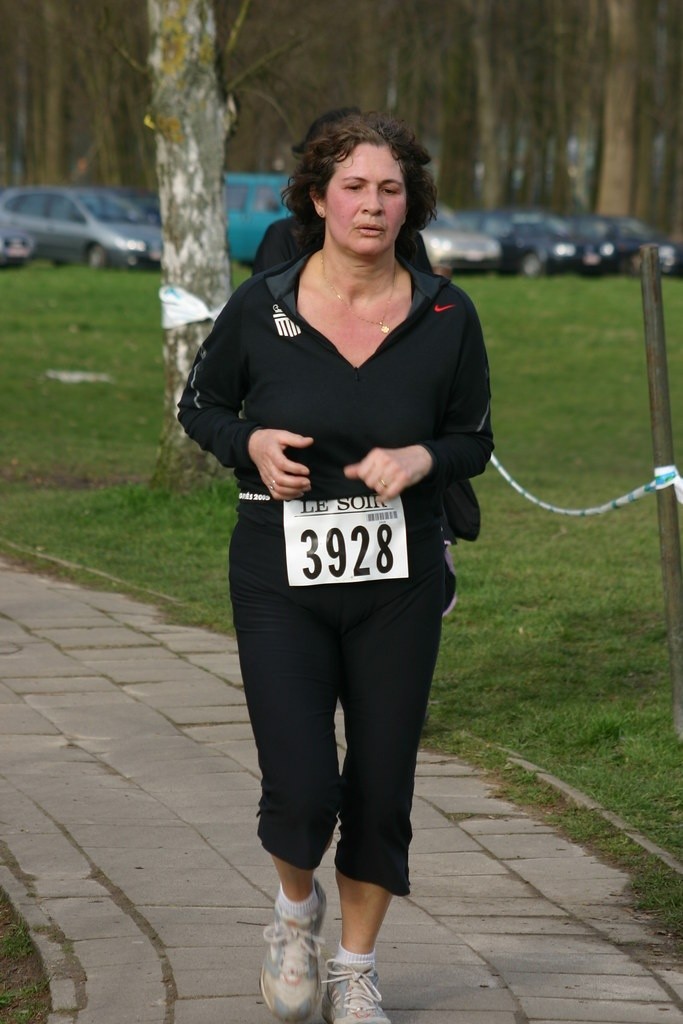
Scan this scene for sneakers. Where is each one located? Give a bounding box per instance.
[259,876,327,1024]
[320,958,391,1024]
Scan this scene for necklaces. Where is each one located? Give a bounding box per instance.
[320,247,398,335]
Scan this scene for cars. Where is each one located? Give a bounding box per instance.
[221,171,295,268]
[0,181,165,273]
[417,202,683,281]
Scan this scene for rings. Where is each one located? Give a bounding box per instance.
[269,479,276,490]
[379,479,387,488]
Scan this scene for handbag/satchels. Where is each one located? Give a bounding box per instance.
[443,479,481,541]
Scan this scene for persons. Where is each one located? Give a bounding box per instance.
[176,107,496,1024]
